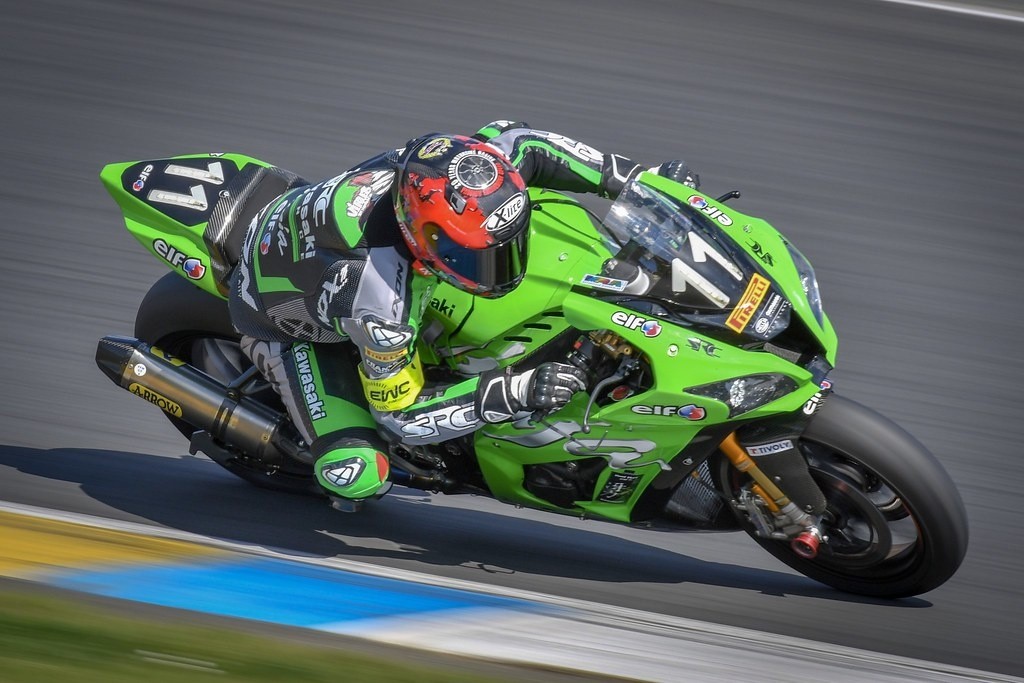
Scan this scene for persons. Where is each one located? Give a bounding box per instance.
[225,120,699,503]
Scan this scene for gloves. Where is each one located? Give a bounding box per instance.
[476,362,588,424]
[600,153,700,207]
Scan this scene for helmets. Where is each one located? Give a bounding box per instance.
[394,133,531,299]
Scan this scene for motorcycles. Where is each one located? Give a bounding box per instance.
[95,152,970,599]
